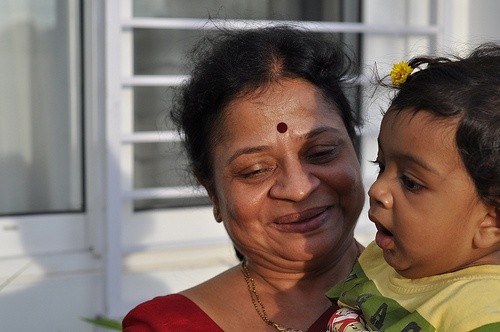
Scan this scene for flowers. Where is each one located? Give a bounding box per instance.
[390,60,413,86]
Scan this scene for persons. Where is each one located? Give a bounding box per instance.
[122,11,367,332]
[325,41,500,332]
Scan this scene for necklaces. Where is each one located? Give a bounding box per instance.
[241,238,362,332]
